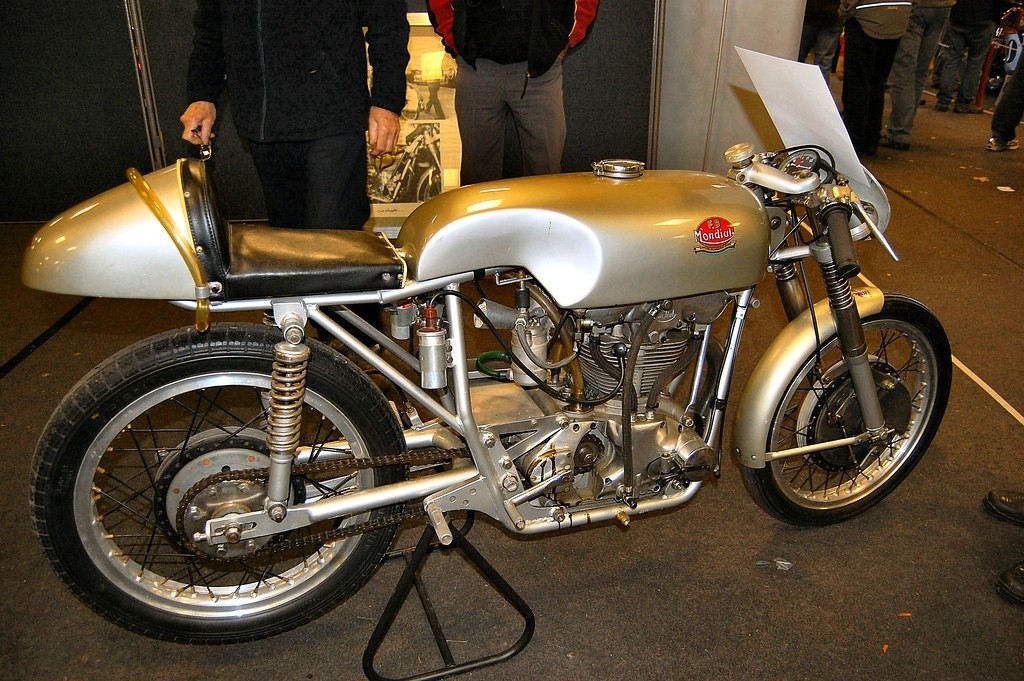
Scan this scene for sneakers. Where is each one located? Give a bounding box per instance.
[985,136,1019,151]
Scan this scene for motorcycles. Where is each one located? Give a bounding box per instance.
[16,146,953,681]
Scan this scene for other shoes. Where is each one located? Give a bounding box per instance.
[953,106,983,113]
[370,343,383,355]
[318,338,350,356]
[879,133,910,151]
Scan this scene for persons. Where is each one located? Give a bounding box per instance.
[798,0,1024,162]
[180,0,412,370]
[981,488,1024,604]
[420,0,600,190]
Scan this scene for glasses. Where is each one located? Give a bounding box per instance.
[190,129,212,161]
